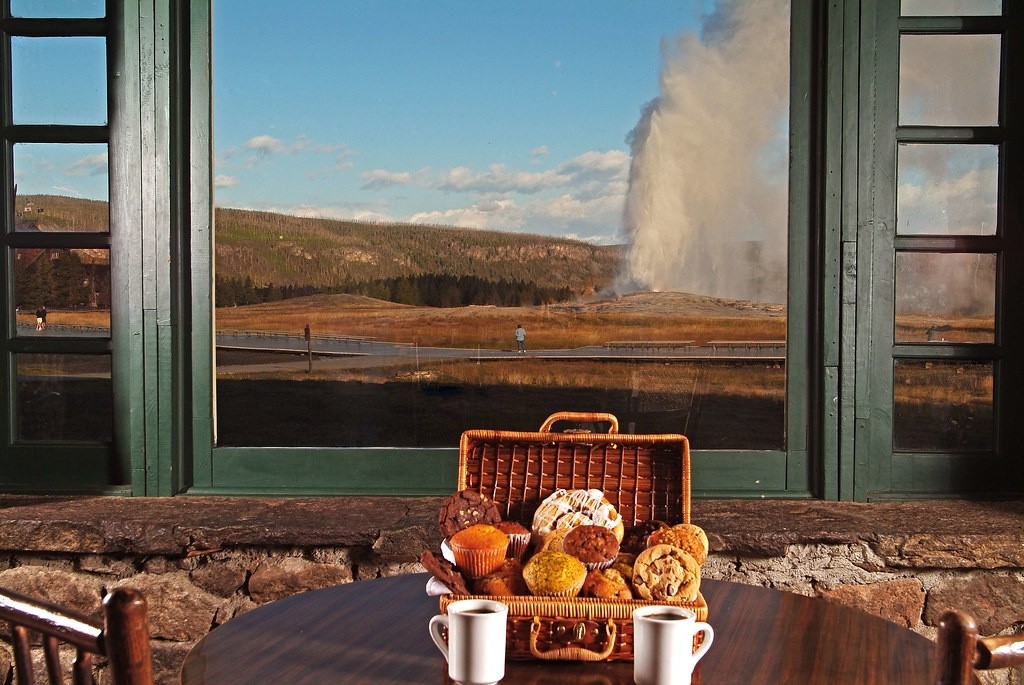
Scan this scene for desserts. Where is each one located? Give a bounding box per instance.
[449,521,637,600]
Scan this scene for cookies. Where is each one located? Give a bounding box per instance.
[624,520,708,603]
[439,489,500,550]
[528,489,623,548]
[420,550,474,595]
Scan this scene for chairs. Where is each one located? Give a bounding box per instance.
[0,586,153,685]
[933,611,1024,685]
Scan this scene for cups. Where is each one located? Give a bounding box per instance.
[632,604,715,685]
[428,599,508,685]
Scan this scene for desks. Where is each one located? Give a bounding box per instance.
[180,572,982,685]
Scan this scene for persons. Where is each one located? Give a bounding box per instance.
[36,305,46,331]
[925,326,936,341]
[515,324,527,354]
